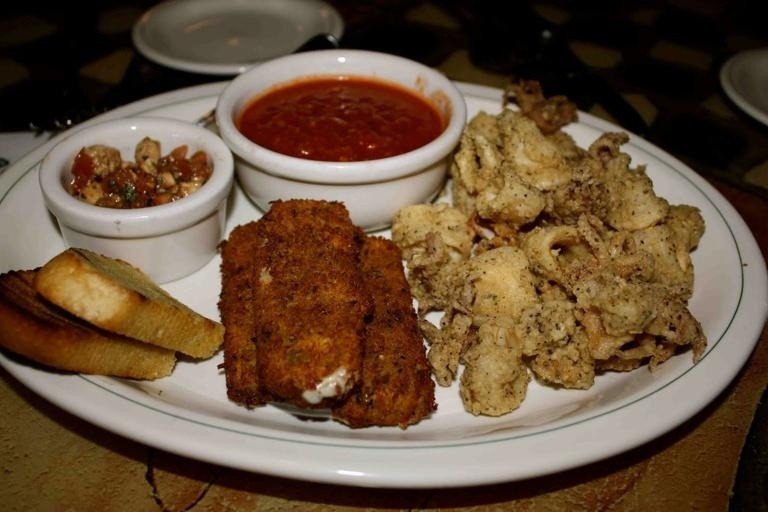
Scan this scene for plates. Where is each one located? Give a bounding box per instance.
[719,44,768,131]
[130,1,354,76]
[1,77,765,492]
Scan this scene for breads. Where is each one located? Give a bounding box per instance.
[1,246,225,382]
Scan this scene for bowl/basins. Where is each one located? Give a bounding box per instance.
[42,116,234,276]
[213,45,469,233]
